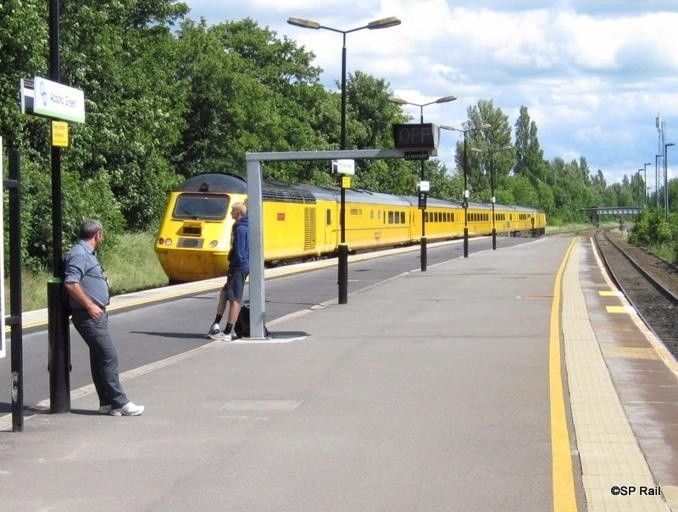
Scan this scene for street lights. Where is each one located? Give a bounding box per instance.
[385,96,457,271]
[438,124,492,258]
[472,146,511,250]
[636,143,674,226]
[286,14,400,302]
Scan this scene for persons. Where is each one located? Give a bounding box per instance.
[205,202,248,342]
[62,217,146,417]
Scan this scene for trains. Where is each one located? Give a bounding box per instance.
[154,171,547,286]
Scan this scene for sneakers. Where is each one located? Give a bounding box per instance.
[208,323,232,341]
[99,402,145,416]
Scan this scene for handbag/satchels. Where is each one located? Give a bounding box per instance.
[234,304,268,339]
